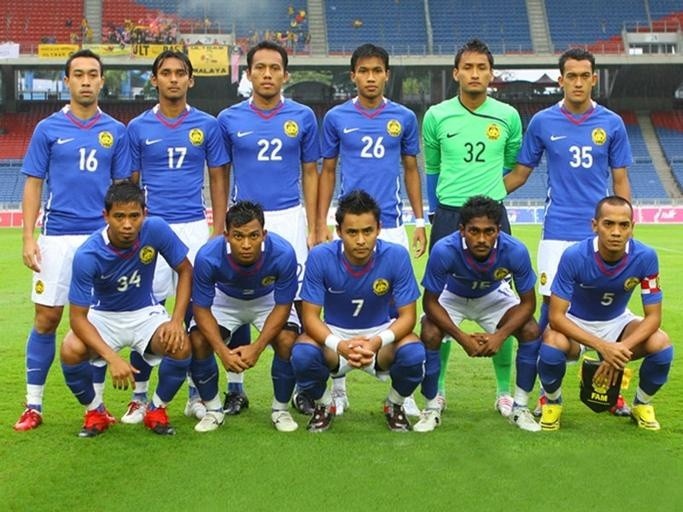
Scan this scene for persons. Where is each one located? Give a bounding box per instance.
[314,44,429,414]
[120,52,230,429]
[188,202,299,434]
[58,180,194,440]
[412,194,544,435]
[216,42,319,415]
[535,195,673,432]
[40,1,313,48]
[289,188,426,434]
[11,48,133,431]
[501,49,634,418]
[421,40,523,420]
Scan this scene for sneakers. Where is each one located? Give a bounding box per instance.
[194,404,226,432]
[437,395,447,411]
[328,394,350,416]
[494,395,514,418]
[412,409,442,433]
[184,399,207,420]
[533,390,544,417]
[121,399,147,424]
[143,404,175,434]
[631,400,660,431]
[78,409,118,436]
[220,390,249,417]
[272,410,299,432]
[292,391,315,414]
[402,395,422,418]
[383,398,412,433]
[607,393,632,417]
[12,401,45,432]
[509,402,542,432]
[305,401,336,432]
[539,396,562,432]
